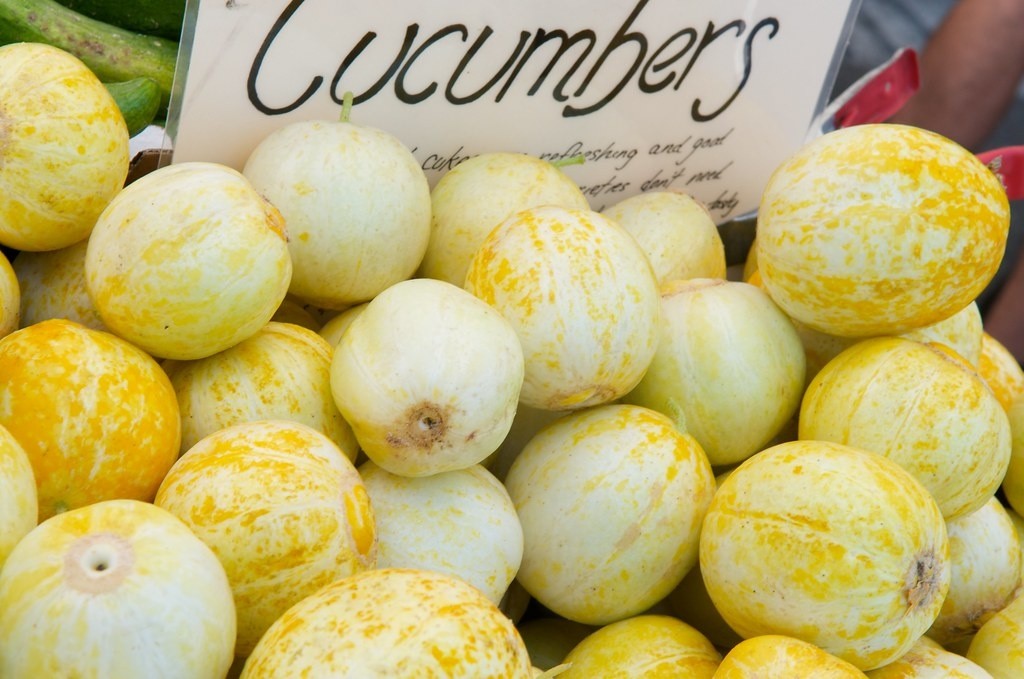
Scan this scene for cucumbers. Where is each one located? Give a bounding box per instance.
[0,0,184,138]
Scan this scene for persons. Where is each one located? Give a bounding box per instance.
[822,0,1024,371]
